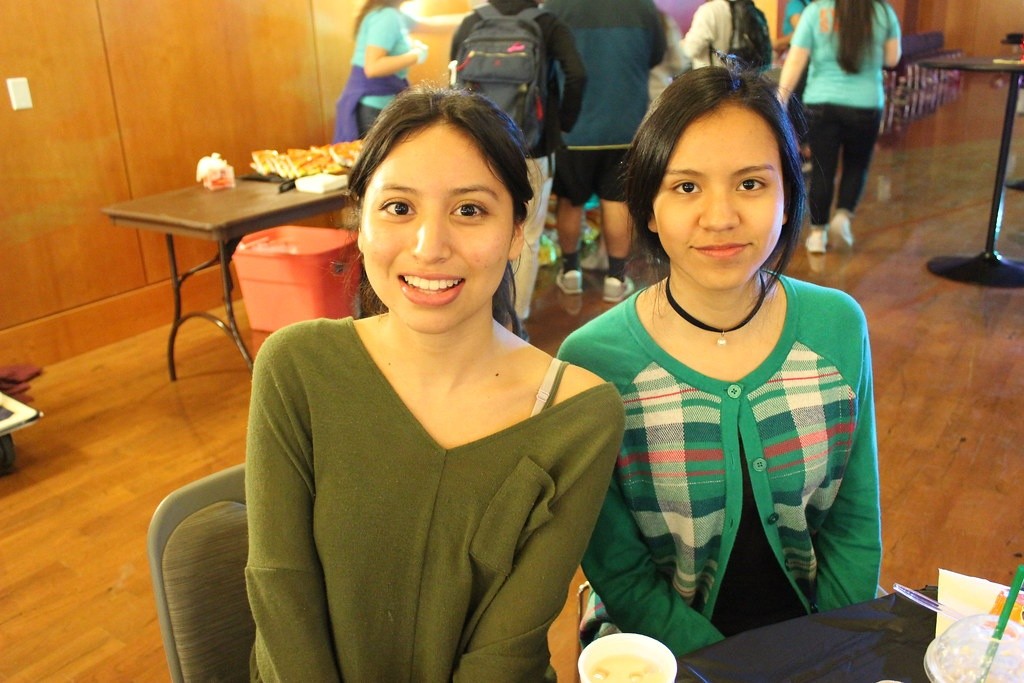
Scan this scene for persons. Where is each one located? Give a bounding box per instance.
[335,0,428,146]
[678,0,772,69]
[536,0,667,302]
[449,0,588,343]
[558,65,883,659]
[778,0,902,252]
[247,93,623,683]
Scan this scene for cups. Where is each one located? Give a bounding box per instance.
[924,613,1024,683]
[577,632,677,683]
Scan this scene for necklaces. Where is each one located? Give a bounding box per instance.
[666,272,765,346]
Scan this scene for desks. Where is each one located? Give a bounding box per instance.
[103,168,358,382]
[918,60,1024,287]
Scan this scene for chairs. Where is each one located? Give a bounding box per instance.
[148,463,254,683]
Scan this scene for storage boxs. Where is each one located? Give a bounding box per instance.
[229,223,363,331]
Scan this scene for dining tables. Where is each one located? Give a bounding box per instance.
[676,583,936,683]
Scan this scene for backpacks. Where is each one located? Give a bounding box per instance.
[456,6,551,152]
[727,0,772,73]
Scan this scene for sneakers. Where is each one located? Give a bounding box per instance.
[554,269,584,295]
[805,228,828,252]
[602,275,636,302]
[831,212,853,246]
